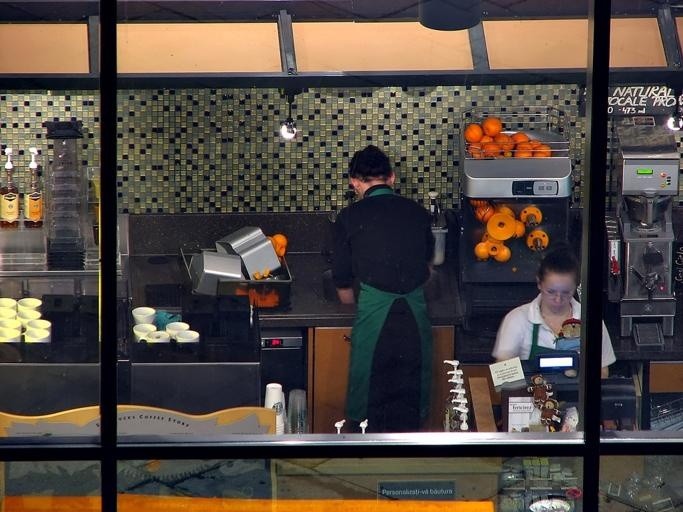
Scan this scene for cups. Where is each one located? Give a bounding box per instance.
[262,382,308,434]
[130,306,200,344]
[0,295,53,345]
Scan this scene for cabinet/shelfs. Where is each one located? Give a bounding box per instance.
[305,325,454,434]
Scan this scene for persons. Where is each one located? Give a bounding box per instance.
[330,146,436,431]
[487,244,618,378]
